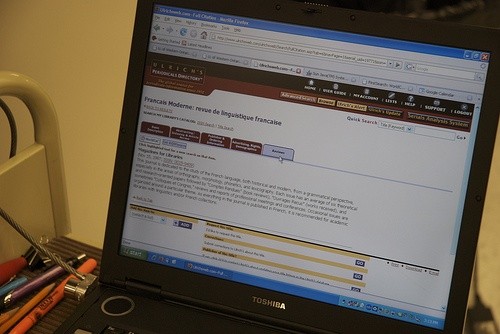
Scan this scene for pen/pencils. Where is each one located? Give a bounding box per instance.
[0,252,98,334]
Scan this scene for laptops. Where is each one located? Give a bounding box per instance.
[50,0,499,334]
